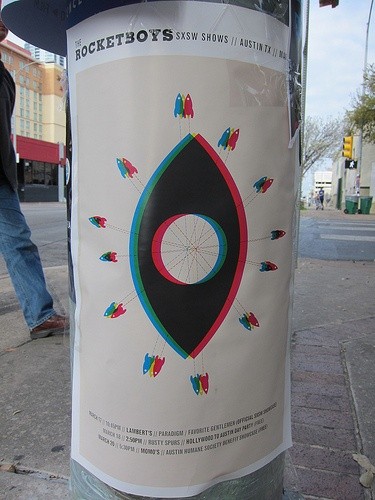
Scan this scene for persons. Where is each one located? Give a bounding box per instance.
[314,187,325,210]
[0,13,70,340]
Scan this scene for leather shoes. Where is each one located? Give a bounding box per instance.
[29,313,70,339]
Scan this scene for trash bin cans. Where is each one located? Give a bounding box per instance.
[343,194,359,214]
[358,195,373,214]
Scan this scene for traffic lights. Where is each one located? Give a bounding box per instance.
[344,158,357,170]
[341,133,357,158]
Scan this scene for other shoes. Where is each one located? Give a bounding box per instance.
[321,207,323,211]
